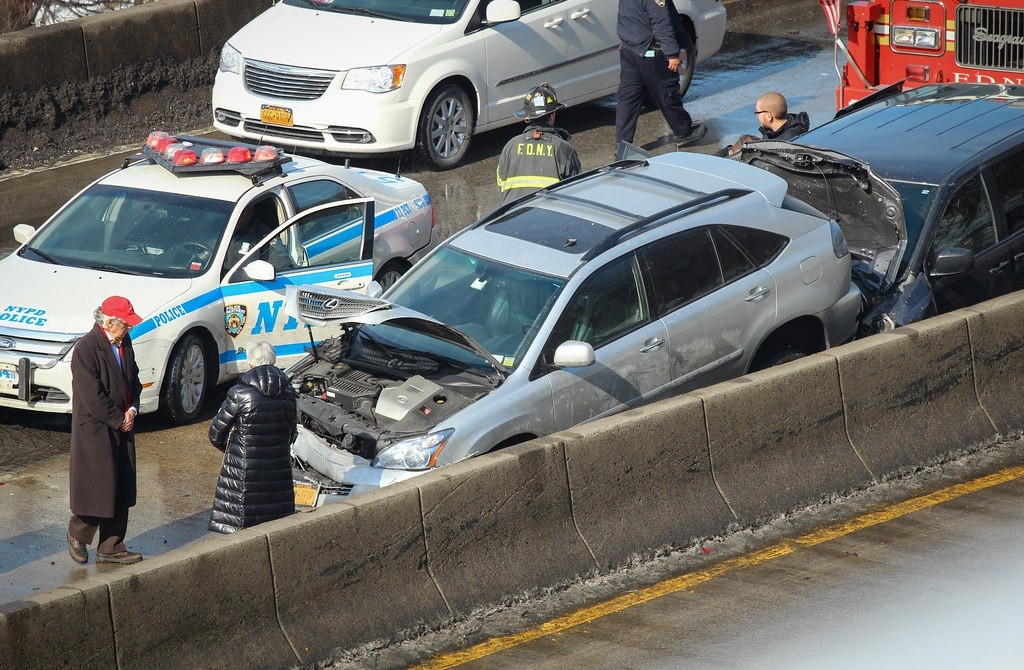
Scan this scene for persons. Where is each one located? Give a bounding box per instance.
[207,341,301,534]
[66,296,143,564]
[713,92,810,157]
[191,205,293,283]
[496,83,582,207]
[614,0,707,156]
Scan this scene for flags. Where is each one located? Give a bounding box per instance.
[819,0,841,33]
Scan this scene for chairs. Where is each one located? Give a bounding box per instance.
[111,207,271,284]
[453,268,640,372]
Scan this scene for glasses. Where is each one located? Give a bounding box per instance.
[114,318,133,331]
[754,110,770,116]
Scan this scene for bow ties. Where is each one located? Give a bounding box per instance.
[110,337,122,347]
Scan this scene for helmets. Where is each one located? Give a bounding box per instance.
[514,83,563,120]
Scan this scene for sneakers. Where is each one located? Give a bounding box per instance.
[67,531,89,564]
[95,550,142,564]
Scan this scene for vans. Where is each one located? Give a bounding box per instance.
[211,0,727,172]
[784,76,1024,314]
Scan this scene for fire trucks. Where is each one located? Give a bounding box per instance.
[836,0,1024,114]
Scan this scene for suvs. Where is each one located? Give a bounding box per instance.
[281,135,868,519]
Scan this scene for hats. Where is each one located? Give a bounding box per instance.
[101,296,143,325]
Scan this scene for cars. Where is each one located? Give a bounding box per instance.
[0,130,439,424]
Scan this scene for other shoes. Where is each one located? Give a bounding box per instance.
[673,123,708,148]
[613,143,622,156]
